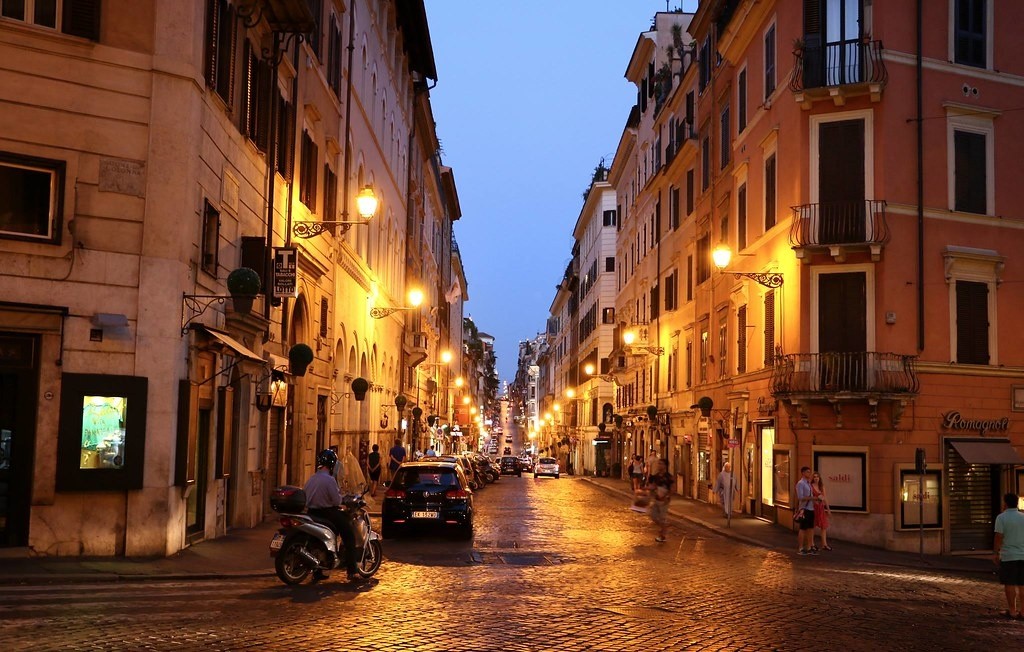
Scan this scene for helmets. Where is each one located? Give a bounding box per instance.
[318,449,337,466]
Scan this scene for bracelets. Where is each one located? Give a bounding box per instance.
[993,551,998,555]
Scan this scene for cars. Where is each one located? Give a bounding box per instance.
[416,400,535,493]
[379,462,478,543]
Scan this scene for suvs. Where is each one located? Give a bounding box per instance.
[532,456,560,479]
[500,455,523,477]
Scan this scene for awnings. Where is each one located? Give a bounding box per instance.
[949,441,1024,470]
[199,328,268,389]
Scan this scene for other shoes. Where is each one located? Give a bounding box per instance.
[1002,610,1015,619]
[1017,612,1024,619]
[798,544,834,555]
[347,574,369,583]
[312,572,329,581]
[655,536,666,542]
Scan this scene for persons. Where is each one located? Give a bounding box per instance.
[795,466,833,555]
[606,409,611,423]
[304,449,370,584]
[627,450,659,491]
[426,445,436,456]
[389,438,406,481]
[713,462,738,516]
[992,492,1024,619]
[366,444,381,497]
[641,459,674,542]
[415,447,423,460]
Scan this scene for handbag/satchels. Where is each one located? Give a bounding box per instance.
[792,508,807,523]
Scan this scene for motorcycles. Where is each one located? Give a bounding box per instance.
[266,480,383,587]
